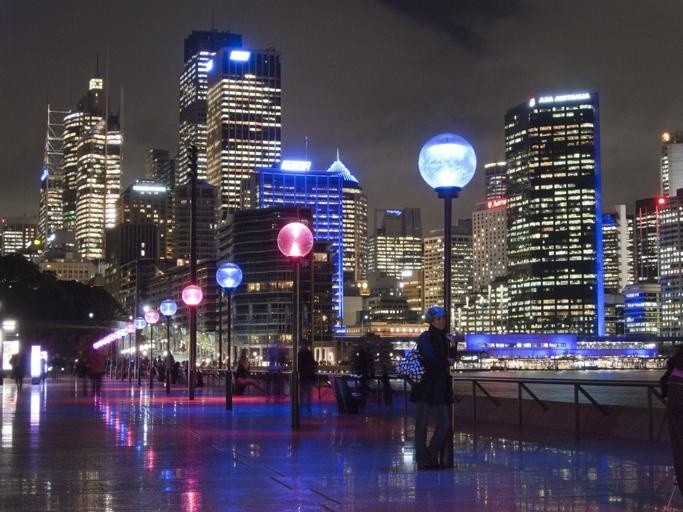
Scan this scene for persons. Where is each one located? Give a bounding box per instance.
[666,349,682,496]
[40,344,189,396]
[9,348,27,392]
[234,348,250,396]
[373,334,399,405]
[299,339,318,413]
[351,330,379,406]
[265,340,285,397]
[409,307,457,470]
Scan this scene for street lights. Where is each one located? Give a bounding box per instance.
[144,309,159,388]
[276,222,314,429]
[181,284,202,400]
[214,263,243,410]
[92,316,146,385]
[417,133,478,468]
[159,299,177,394]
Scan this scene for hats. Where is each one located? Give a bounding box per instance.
[425,307,447,322]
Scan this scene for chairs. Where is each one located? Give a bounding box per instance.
[231,369,245,395]
[328,375,362,414]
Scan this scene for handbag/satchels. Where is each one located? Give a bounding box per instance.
[396,350,428,386]
